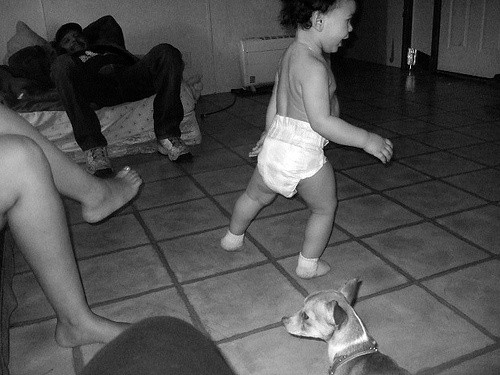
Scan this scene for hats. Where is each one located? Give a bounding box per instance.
[55,22,83,42]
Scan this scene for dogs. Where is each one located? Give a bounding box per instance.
[281,276,412,375]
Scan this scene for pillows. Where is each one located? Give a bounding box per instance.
[3,20,45,64]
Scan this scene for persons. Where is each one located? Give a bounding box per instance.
[77,316,235,375]
[51,23,191,179]
[220,0,393,280]
[0,104,142,349]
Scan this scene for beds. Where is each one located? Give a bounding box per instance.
[16,65,205,165]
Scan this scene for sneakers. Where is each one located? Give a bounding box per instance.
[158,137,194,159]
[83,145,118,177]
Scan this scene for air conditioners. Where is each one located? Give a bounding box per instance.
[239,35,295,92]
